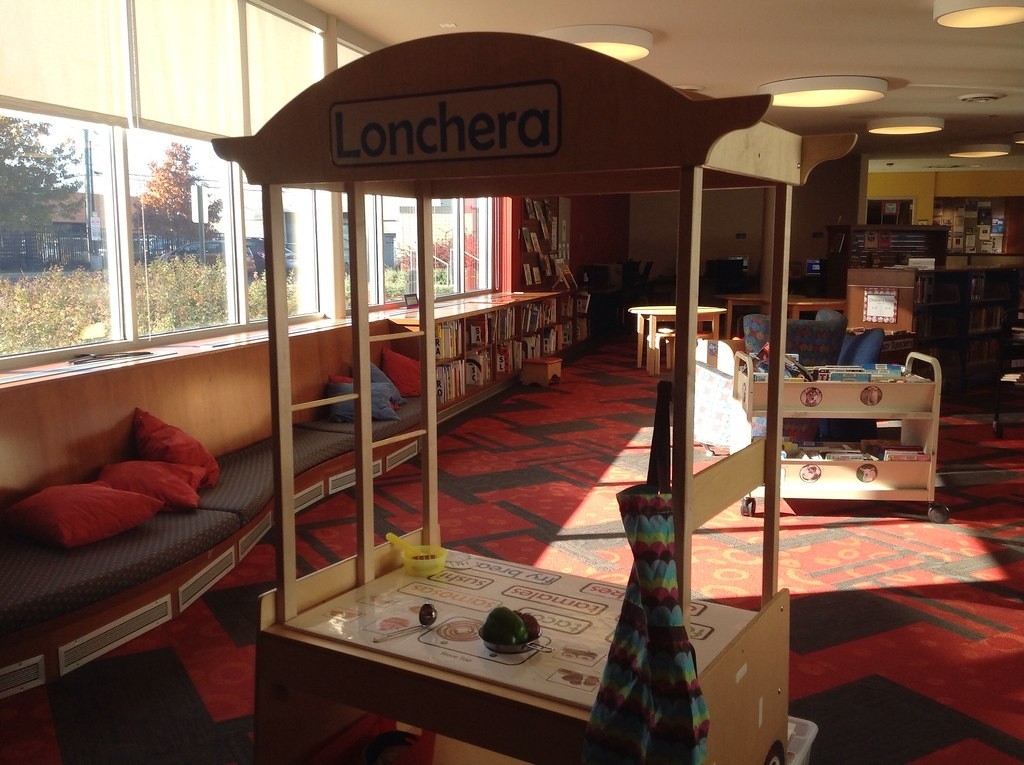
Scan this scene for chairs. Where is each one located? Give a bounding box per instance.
[615,260,654,324]
[729,306,884,447]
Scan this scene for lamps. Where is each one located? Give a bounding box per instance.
[866,117,945,135]
[533,25,652,63]
[948,144,1012,158]
[932,0,1024,30]
[757,75,888,110]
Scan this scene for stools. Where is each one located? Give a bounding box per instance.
[522,357,563,388]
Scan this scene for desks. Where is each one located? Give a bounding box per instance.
[628,306,728,373]
[712,294,847,338]
[587,285,621,331]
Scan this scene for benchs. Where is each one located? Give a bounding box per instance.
[0,397,425,699]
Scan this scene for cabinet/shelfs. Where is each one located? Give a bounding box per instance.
[740,267,1024,524]
[398,289,564,422]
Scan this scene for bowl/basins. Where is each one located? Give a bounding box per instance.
[401,545,445,578]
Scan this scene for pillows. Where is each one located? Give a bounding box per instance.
[131,406,219,491]
[1,478,164,549]
[325,360,409,423]
[90,460,208,512]
[379,345,422,397]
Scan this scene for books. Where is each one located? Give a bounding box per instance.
[434,290,591,404]
[738,342,933,461]
